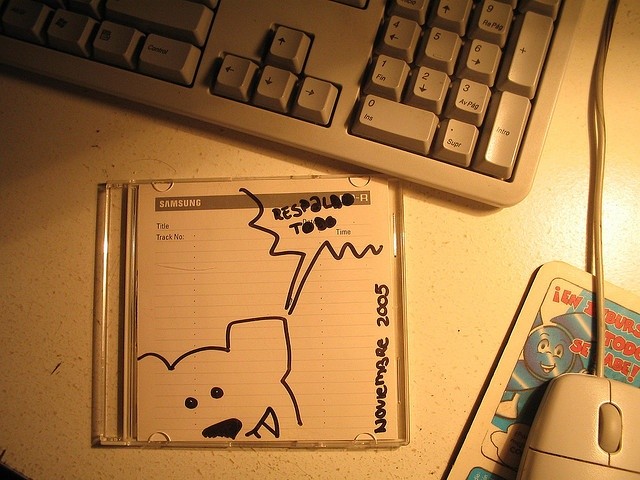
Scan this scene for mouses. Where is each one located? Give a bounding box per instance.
[516,374,639,479]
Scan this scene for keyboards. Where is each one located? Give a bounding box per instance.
[0,0,587,207]
